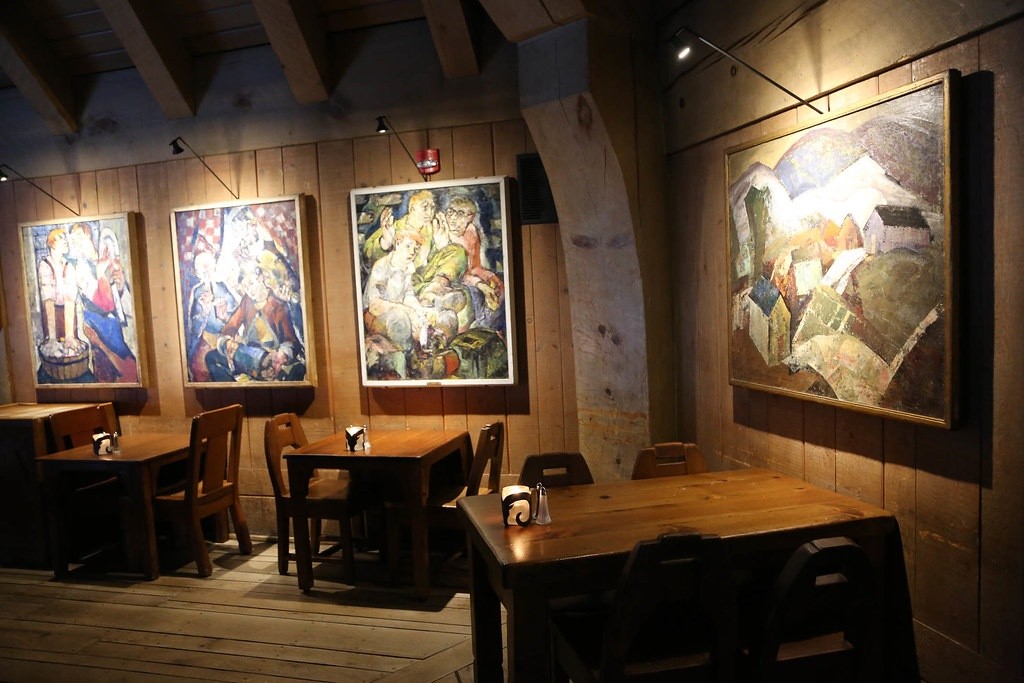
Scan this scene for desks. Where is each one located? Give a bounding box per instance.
[456,467,919,683]
[34,431,231,582]
[282,427,475,602]
[0,399,116,572]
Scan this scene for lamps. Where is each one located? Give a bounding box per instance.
[667,23,825,117]
[0,163,79,216]
[374,112,428,182]
[169,136,242,202]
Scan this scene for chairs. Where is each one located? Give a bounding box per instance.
[263,412,504,591]
[50,401,254,579]
[518,441,885,683]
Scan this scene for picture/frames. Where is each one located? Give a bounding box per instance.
[169,192,320,390]
[721,69,971,436]
[15,211,150,391]
[350,173,521,392]
